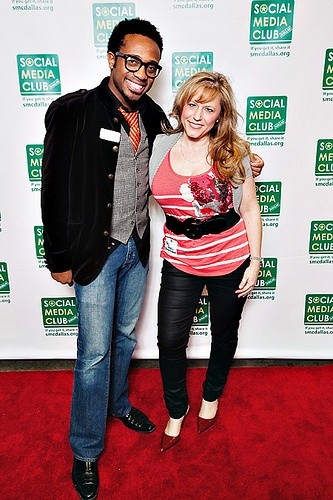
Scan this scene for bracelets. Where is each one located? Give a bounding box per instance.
[250,257,261,262]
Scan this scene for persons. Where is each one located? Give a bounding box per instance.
[148,71,263,452]
[41,19,264,500]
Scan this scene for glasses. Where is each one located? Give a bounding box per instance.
[115,51,163,79]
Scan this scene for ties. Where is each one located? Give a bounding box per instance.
[118,106,141,152]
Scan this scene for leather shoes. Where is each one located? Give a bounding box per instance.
[108,407,156,433]
[72,456,98,500]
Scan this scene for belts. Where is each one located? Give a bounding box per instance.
[166,208,240,240]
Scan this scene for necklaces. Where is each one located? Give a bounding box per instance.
[179,137,207,163]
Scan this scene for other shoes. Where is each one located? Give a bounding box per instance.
[160,405,190,453]
[196,404,219,433]
